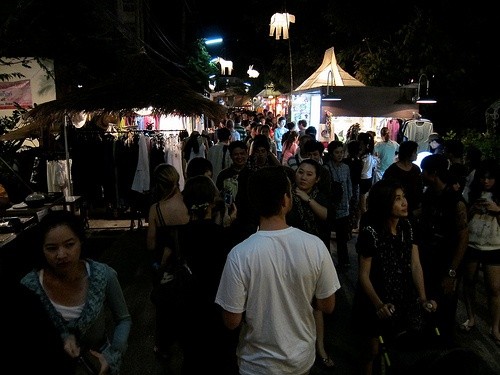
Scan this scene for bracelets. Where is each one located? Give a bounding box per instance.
[307,197,313,203]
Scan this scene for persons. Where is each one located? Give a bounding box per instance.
[217,168,341,375]
[11,210,130,374]
[289,160,336,366]
[140,104,500,343]
[351,177,439,366]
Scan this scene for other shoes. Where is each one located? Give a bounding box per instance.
[321,354,334,369]
